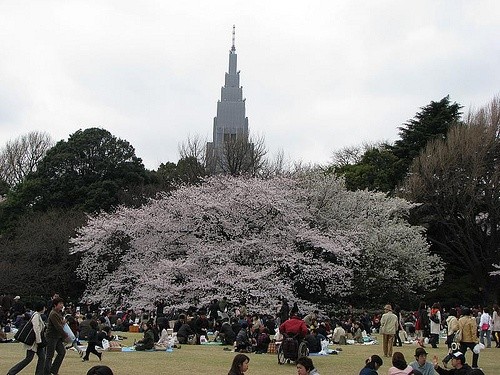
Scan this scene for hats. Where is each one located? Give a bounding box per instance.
[449,352,465,362]
[383,305,392,310]
[414,348,429,356]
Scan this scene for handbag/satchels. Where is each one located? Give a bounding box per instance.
[481,324,489,331]
[154,343,167,351]
[431,310,439,323]
[455,330,463,341]
[473,342,485,354]
[14,313,39,346]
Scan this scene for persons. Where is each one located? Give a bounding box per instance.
[409,348,433,375]
[407,369,422,374]
[0,291,499,366]
[47,298,74,375]
[297,356,320,375]
[7,300,46,375]
[433,351,476,375]
[228,354,251,375]
[87,365,113,375]
[379,304,399,357]
[388,351,414,375]
[359,354,384,374]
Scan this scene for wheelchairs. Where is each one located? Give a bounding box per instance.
[277,339,306,366]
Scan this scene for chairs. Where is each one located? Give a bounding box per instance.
[269,335,279,354]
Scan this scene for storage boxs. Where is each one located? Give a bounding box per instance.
[129,326,139,333]
[345,338,354,345]
[108,346,122,352]
[5,332,16,340]
[362,336,370,342]
[208,335,216,341]
[167,328,173,335]
